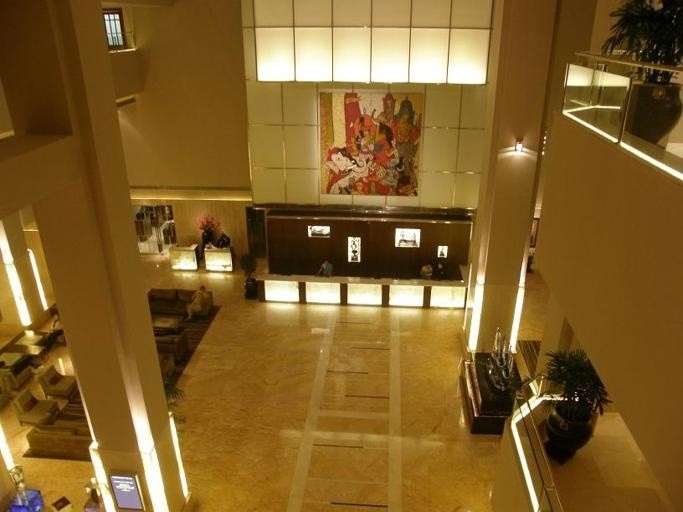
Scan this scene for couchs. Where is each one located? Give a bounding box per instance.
[26,418,92,460]
[146,288,212,318]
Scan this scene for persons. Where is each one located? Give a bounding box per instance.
[186,286,207,321]
[421,261,435,278]
[437,261,447,279]
[317,259,334,276]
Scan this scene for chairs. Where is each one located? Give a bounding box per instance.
[0,367,77,426]
[155,331,187,364]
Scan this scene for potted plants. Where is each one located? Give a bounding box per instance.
[598,0,682,150]
[538,347,613,451]
[239,253,258,299]
